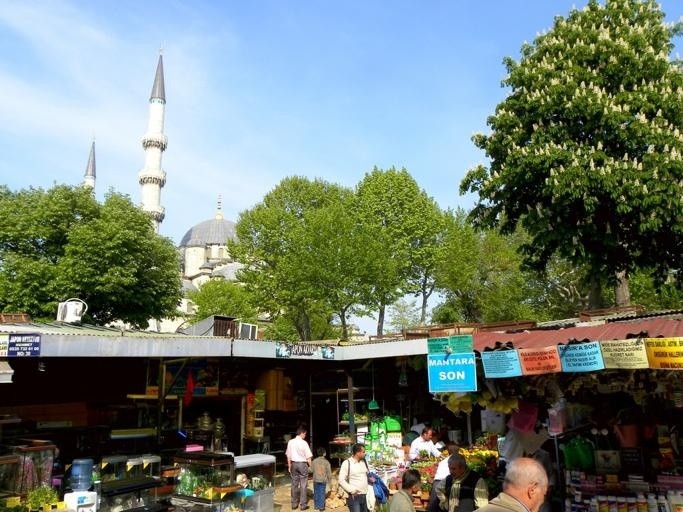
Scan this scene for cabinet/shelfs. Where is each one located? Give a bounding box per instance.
[62,455,162,512]
[329,387,370,467]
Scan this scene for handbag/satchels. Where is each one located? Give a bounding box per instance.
[334,459,350,507]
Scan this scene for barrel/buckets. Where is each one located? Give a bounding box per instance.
[253,426,264,438]
[447,429,462,445]
[69,458,93,492]
[548,406,567,433]
[214,417,223,434]
[201,412,211,428]
[666,489,683,512]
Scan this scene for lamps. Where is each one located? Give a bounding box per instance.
[38,362,45,371]
[368,378,379,410]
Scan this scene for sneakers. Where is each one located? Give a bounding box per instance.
[292,504,298,510]
[300,505,310,510]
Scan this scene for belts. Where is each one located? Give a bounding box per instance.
[293,461,307,464]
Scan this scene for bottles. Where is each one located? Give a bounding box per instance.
[197,411,224,433]
[589,489,683,512]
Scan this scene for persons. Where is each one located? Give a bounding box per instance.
[310,448,332,512]
[408,426,445,464]
[472,457,548,512]
[388,468,421,512]
[337,443,376,512]
[284,426,313,511]
[429,444,459,512]
[485,455,500,477]
[434,454,489,512]
